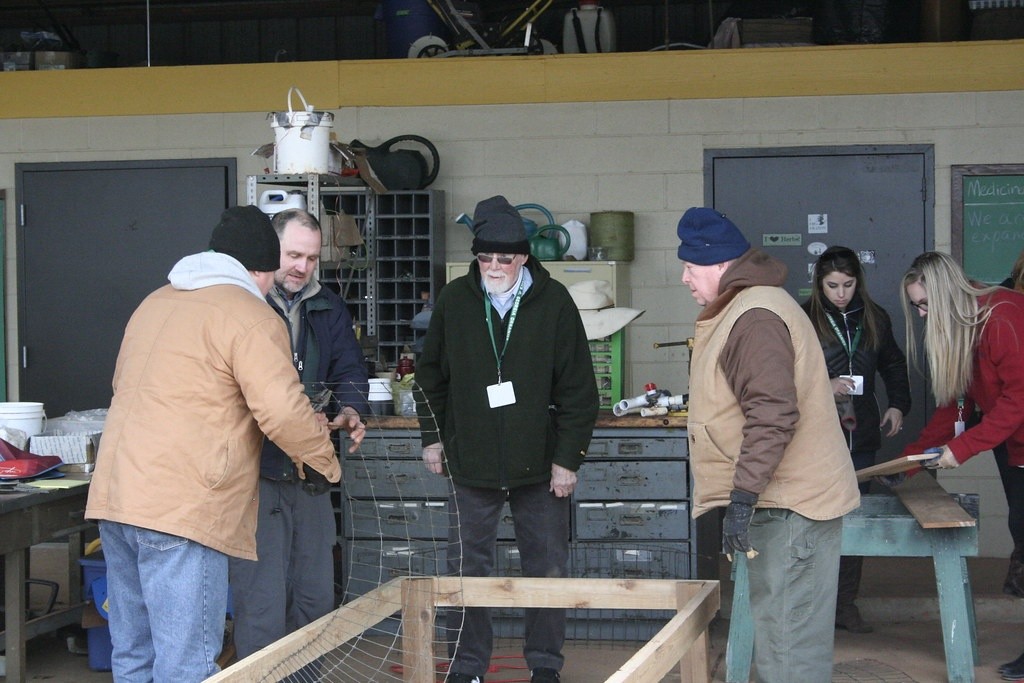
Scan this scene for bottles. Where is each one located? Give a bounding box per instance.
[396,356,414,381]
[559,218,587,261]
[563,0,617,53]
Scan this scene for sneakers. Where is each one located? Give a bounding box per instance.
[445,672,484,683]
[530,667,560,683]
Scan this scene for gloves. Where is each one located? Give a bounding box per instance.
[834,395,857,430]
[722,487,759,562]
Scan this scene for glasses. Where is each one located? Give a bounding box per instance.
[477,253,516,265]
[820,249,855,262]
[910,300,928,311]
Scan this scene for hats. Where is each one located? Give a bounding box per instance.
[471,195,530,256]
[209,205,280,271]
[677,207,751,265]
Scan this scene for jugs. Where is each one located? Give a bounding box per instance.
[453,202,571,261]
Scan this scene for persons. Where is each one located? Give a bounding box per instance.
[410,195,599,683]
[900,251,1024,680]
[799,245,911,633]
[676,206,861,683]
[991,250,1024,600]
[225,208,369,683]
[83,203,340,683]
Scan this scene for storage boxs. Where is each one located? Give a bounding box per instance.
[26,429,102,473]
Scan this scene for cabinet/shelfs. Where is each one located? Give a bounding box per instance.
[246,173,445,379]
[338,412,722,643]
[445,258,628,410]
[0,471,96,683]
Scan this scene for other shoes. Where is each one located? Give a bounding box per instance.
[1003,559,1024,598]
[997,653,1024,681]
[835,606,873,633]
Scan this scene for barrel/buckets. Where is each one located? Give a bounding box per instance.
[374,0,447,58]
[368,377,390,401]
[587,211,635,261]
[78,536,113,672]
[270,85,334,175]
[0,402,48,443]
